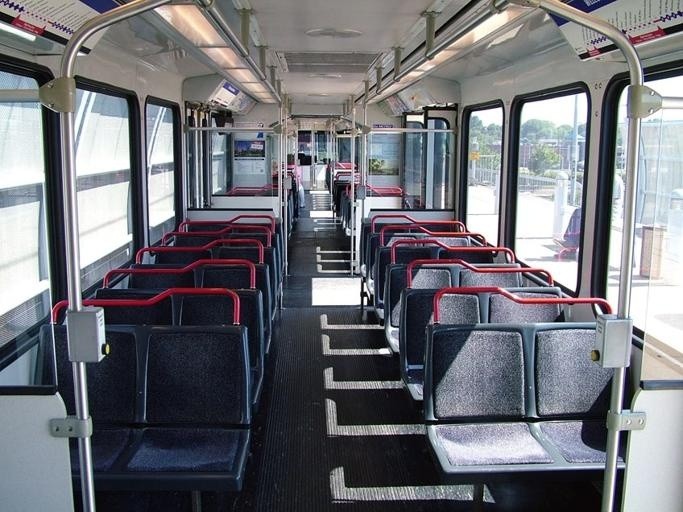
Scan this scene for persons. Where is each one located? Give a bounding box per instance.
[553,162,625,250]
[287,153,306,209]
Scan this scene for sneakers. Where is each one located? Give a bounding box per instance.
[553,238,571,249]
